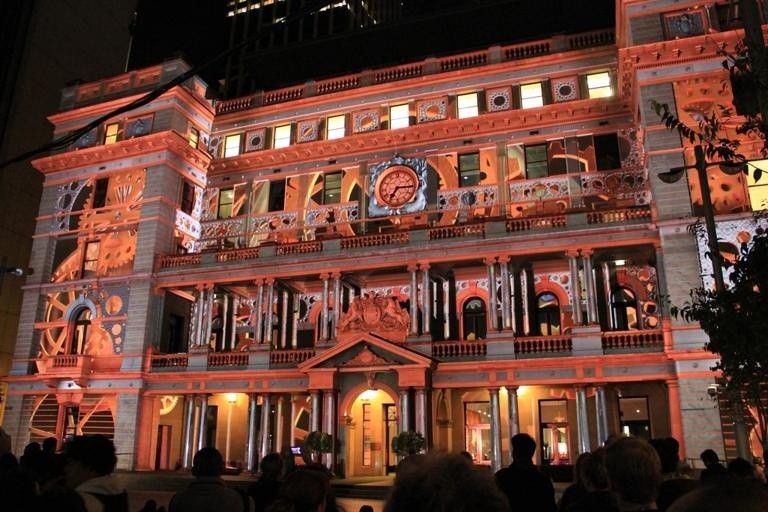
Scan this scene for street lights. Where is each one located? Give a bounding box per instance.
[656,145,757,469]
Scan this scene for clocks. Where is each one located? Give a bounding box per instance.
[364,156,427,219]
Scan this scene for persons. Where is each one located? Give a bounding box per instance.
[0,427,130,512]
[384,430,767,512]
[142,446,374,512]
